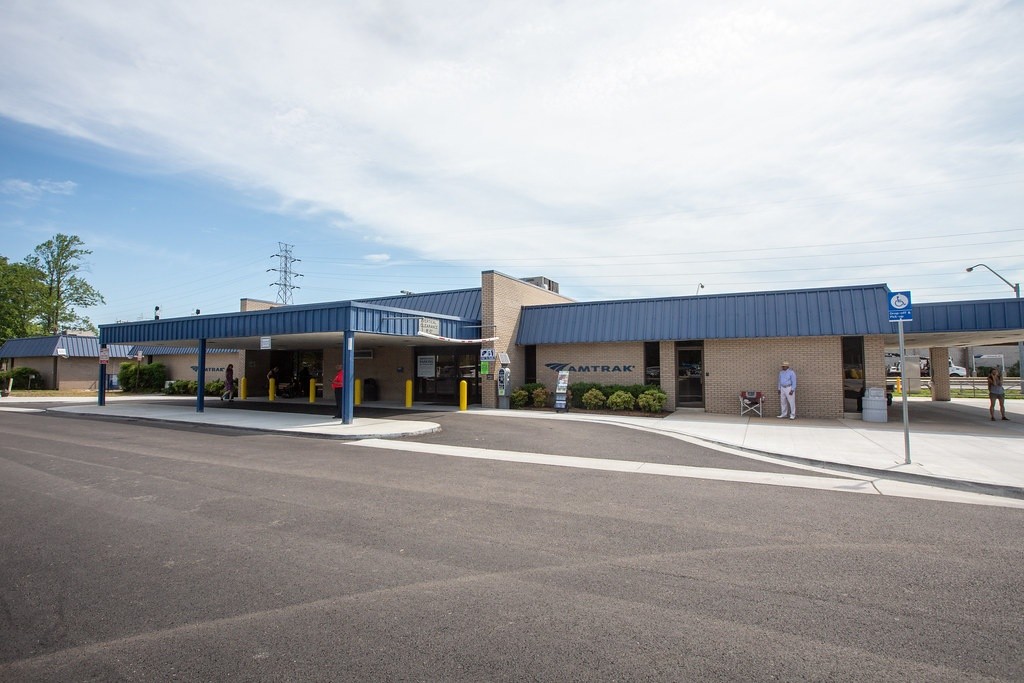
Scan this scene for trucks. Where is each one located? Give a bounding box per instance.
[887,356,966,377]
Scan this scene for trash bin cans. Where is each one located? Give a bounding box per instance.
[364,378,381,401]
[861,387,888,422]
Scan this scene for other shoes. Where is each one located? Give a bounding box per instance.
[221,396,224,401]
[229,400,234,402]
[777,415,788,419]
[991,417,995,421]
[790,415,795,420]
[1002,416,1010,420]
[333,416,342,418]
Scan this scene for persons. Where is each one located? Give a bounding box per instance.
[777,362,797,420]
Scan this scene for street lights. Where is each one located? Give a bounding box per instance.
[966,264,1024,394]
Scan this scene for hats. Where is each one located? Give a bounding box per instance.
[782,361,789,366]
